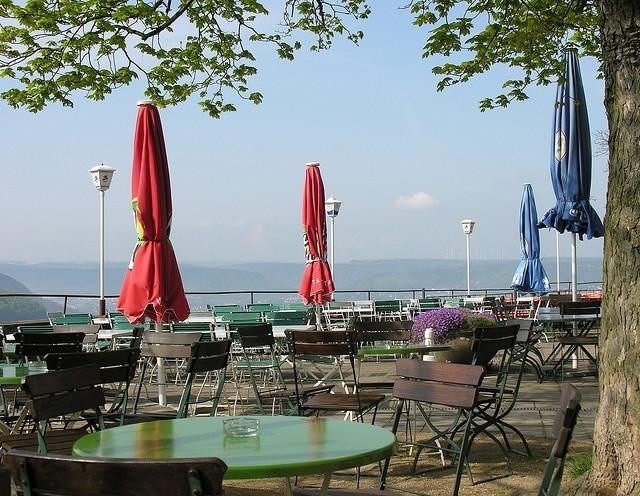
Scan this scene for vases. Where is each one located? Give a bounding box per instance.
[433,336,498,364]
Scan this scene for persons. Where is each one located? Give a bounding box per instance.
[500,295,508,305]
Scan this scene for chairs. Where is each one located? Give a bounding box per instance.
[345,320,414,446]
[354,300,373,322]
[0,449,228,496]
[114,316,151,331]
[18,325,54,334]
[453,319,533,466]
[376,300,400,322]
[236,323,334,418]
[108,332,135,350]
[273,304,286,311]
[550,294,584,360]
[247,304,271,312]
[0,349,141,454]
[81,333,203,430]
[224,322,283,387]
[484,296,496,312]
[560,302,601,381]
[21,364,105,454]
[421,299,441,310]
[538,383,582,496]
[265,311,308,326]
[108,312,123,328]
[53,324,100,351]
[284,328,386,490]
[177,338,234,419]
[149,322,182,386]
[502,301,545,365]
[230,312,264,361]
[491,301,544,385]
[171,323,219,387]
[464,298,484,311]
[50,313,92,326]
[288,304,307,310]
[412,324,521,473]
[442,297,460,308]
[328,301,354,327]
[381,358,512,496]
[449,328,473,340]
[396,300,420,320]
[0,332,86,416]
[212,304,242,351]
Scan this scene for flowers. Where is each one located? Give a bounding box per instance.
[411,307,497,344]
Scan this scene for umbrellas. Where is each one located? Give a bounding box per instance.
[536,46,604,371]
[510,183,551,309]
[116,101,189,406]
[298,163,335,331]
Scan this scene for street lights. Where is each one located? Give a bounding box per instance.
[461,218,475,297]
[326,196,343,301]
[88,164,116,316]
